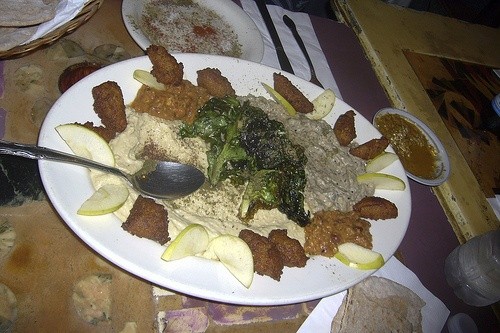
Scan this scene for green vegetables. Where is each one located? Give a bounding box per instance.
[176,94,311,228]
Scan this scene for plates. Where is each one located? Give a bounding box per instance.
[371,107,451,186]
[35,52,412,307]
[121,0,264,64]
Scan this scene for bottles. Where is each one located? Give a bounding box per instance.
[444,229,500,307]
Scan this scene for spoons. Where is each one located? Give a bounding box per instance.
[0,139,205,200]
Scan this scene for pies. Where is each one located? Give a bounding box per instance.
[328,276,426,333]
[0,0,57,52]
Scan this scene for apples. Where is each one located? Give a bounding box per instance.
[208,234,254,287]
[55,125,115,176]
[306,89,336,120]
[77,183,130,216]
[365,152,398,173]
[262,82,296,116]
[162,224,210,261]
[334,243,385,270]
[356,173,405,190]
